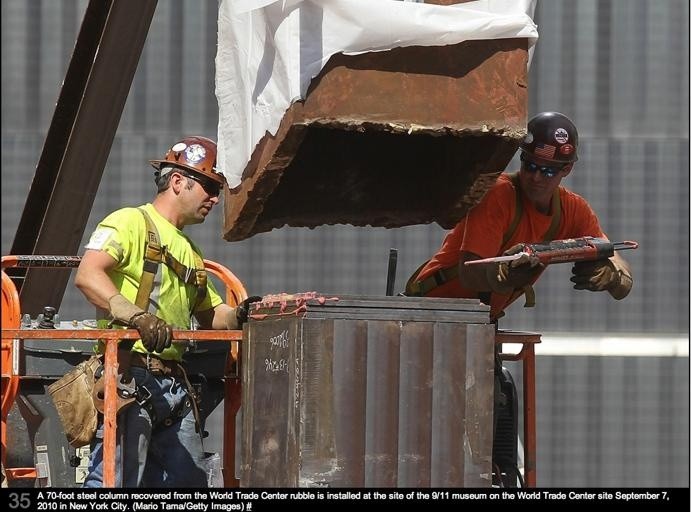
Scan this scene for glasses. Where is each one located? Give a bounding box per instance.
[520,152,562,178]
[170,171,219,197]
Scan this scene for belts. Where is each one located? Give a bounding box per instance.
[131,351,171,375]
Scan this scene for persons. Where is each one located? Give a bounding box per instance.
[75,137,262,487]
[402,112,633,330]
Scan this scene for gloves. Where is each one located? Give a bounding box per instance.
[570,259,633,300]
[486,243,545,295]
[108,294,172,353]
[225,296,262,330]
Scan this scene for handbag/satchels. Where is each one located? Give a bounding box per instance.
[45,355,104,449]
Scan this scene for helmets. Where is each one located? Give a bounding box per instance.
[519,112,579,164]
[149,135,224,184]
